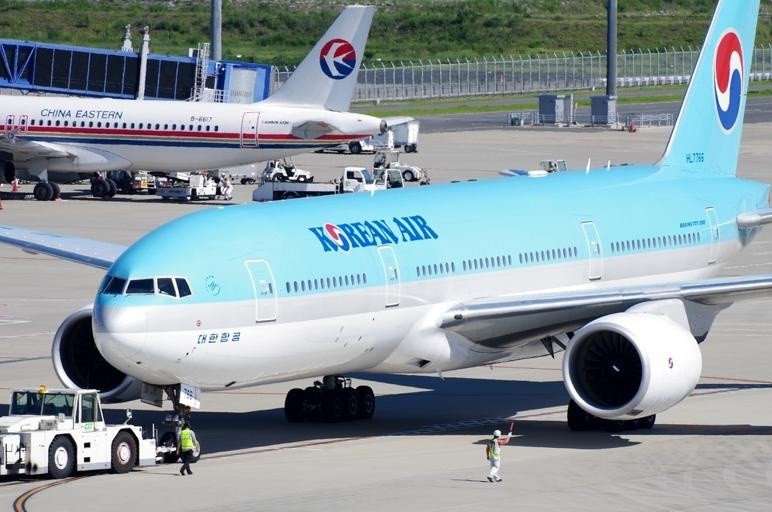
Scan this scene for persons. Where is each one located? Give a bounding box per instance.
[486,430,512,482]
[212,173,233,200]
[176,421,196,475]
[11,176,19,199]
[281,158,295,177]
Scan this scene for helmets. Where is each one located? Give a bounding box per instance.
[493,430,502,437]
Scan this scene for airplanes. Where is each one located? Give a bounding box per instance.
[0,5,414,200]
[0,1,771,430]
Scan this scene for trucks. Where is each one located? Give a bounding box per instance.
[0,387,158,477]
[106,164,228,200]
[252,151,422,202]
[321,120,420,153]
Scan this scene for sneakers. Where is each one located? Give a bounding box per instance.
[487,477,502,482]
